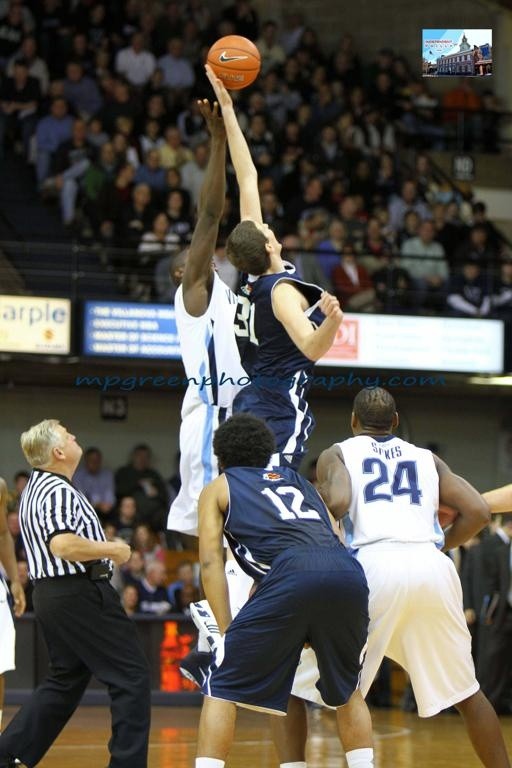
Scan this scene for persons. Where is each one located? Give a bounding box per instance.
[0,476,27,731]
[0,441,511,714]
[178,63,346,472]
[194,414,377,768]
[270,386,511,767]
[1,1,511,320]
[163,98,251,690]
[0,418,153,768]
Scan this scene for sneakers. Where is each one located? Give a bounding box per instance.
[189,599,221,654]
[179,643,213,688]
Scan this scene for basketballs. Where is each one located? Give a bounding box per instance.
[207,36,261,90]
